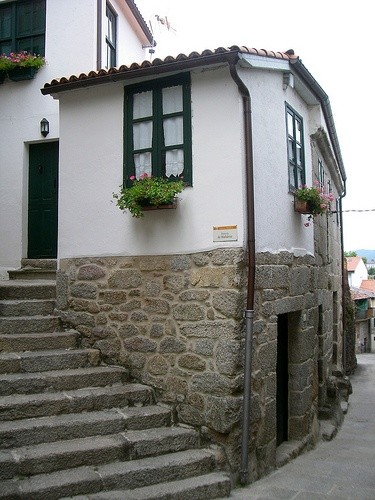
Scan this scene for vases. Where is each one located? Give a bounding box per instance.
[8,66,40,82]
[293,195,313,215]
[0,69,7,85]
[137,198,178,210]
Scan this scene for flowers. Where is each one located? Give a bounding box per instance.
[0,50,46,72]
[109,171,189,220]
[292,179,336,228]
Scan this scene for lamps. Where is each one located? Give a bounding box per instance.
[40,117,50,138]
